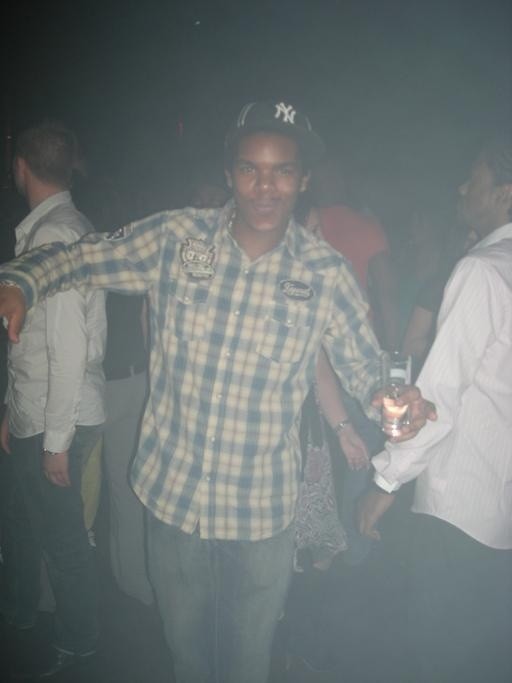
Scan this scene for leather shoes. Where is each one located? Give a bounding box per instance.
[37,645,97,677]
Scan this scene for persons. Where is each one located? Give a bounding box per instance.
[98,294,160,619]
[4,97,435,683]
[354,144,512,683]
[294,191,480,577]
[3,118,108,680]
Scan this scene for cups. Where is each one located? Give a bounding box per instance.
[376,348,414,437]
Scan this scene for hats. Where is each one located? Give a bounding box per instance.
[224,97,329,161]
